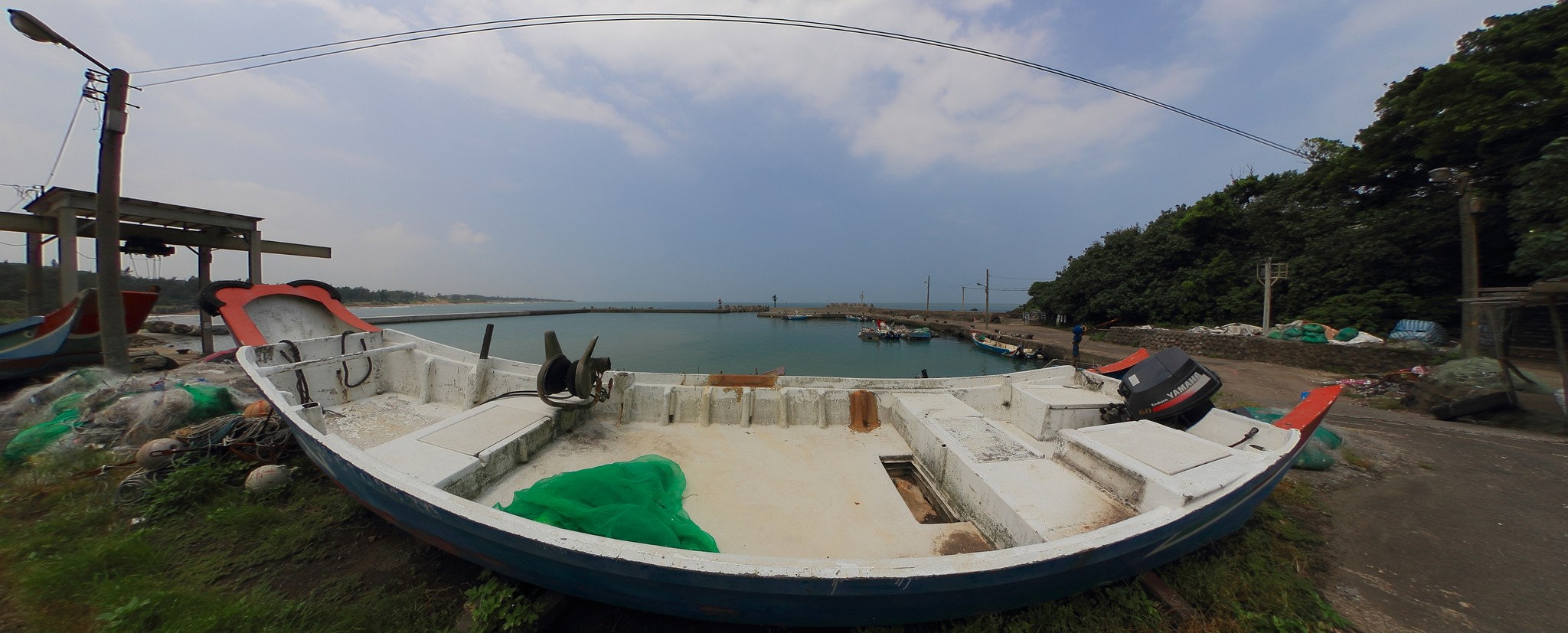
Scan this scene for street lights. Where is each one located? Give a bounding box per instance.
[5,7,132,375]
[924,281,930,311]
[976,283,989,330]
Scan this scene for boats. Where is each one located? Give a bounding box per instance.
[899,323,933,338]
[778,309,813,320]
[844,313,868,321]
[0,288,159,390]
[876,319,903,339]
[970,330,1043,360]
[857,326,882,339]
[200,278,1346,633]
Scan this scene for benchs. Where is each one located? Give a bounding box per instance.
[888,389,1137,550]
[366,390,593,498]
